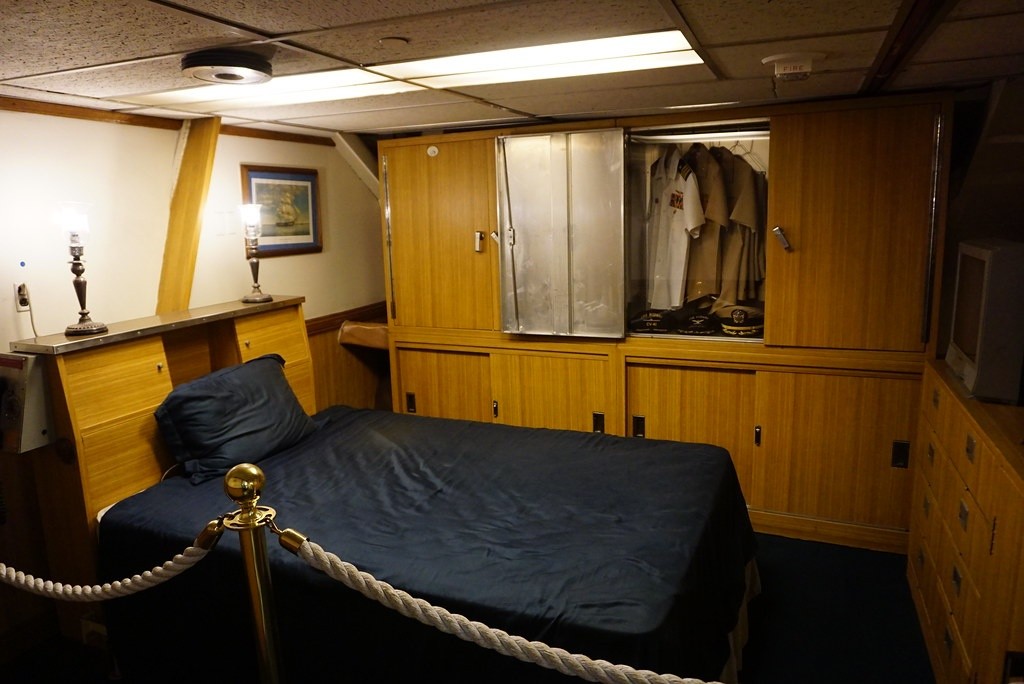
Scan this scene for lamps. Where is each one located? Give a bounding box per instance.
[60,203,108,336]
[239,203,273,303]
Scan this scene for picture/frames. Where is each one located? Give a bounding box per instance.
[240,164,324,259]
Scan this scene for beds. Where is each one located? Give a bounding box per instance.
[91,402,765,684]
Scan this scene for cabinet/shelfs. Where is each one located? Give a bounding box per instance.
[908,361,1024,684]
[613,345,925,563]
[388,332,618,438]
[363,131,506,342]
[758,108,937,350]
[7,290,318,552]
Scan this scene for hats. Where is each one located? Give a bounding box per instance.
[629,309,670,333]
[716,305,765,337]
[677,311,720,335]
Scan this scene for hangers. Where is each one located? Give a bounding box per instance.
[664,142,768,178]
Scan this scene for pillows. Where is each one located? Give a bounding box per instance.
[152,353,315,488]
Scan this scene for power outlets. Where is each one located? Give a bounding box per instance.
[12,281,31,312]
